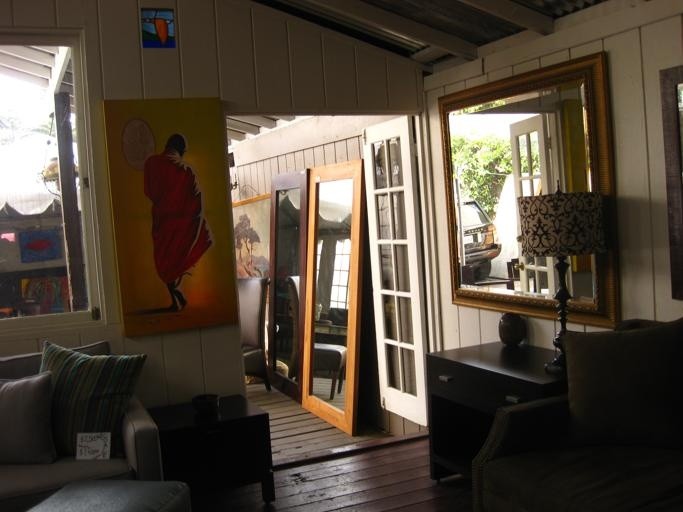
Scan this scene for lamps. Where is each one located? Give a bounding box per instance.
[37,111,80,198]
[516,180,608,374]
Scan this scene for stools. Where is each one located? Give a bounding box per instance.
[26,479,192,512]
[314,343,347,400]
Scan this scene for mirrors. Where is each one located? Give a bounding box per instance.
[438,51,621,330]
[301,158,366,437]
[268,168,310,404]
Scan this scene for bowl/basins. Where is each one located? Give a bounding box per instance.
[191,392,222,413]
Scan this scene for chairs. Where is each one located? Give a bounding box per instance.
[472,317,683,512]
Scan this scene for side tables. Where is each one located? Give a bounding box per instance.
[146,394,275,504]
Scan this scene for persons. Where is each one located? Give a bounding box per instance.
[144,133,213,314]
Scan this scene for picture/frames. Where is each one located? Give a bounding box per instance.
[659,65,683,300]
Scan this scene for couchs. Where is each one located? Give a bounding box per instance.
[0,340,165,512]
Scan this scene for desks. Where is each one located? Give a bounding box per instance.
[426,342,566,484]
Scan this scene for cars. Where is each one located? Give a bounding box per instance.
[458,197,503,282]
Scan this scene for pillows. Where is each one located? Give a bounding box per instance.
[39,340,147,458]
[0,371,59,464]
[563,317,683,449]
